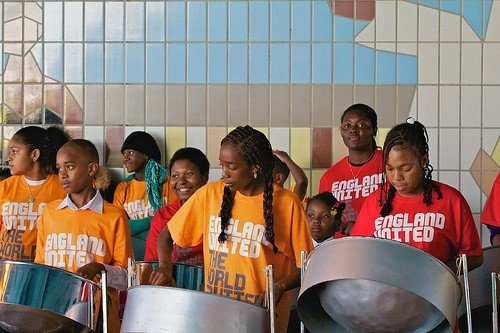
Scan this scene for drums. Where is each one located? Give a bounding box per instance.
[130,258,204,293]
[452,243,500,333]
[119,282,272,333]
[0,260,111,333]
[295,235,463,333]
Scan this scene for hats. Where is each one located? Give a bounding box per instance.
[120,131,162,164]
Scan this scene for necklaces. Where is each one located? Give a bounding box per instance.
[24,175,49,203]
[348,153,373,179]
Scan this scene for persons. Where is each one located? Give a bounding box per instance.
[319,102,387,237]
[305,191,346,247]
[479,173,500,247]
[0,125,313,333]
[351,120,484,276]
